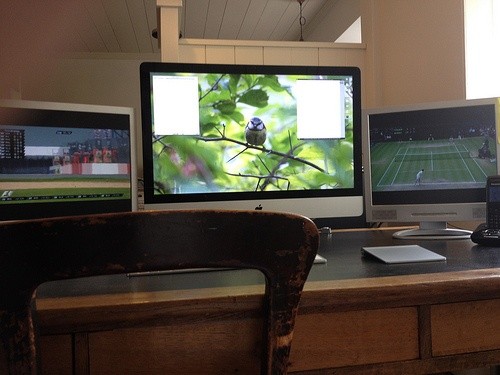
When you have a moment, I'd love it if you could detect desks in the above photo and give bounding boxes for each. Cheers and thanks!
[34,225,500,375]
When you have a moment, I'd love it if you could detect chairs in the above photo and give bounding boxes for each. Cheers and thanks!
[0,209,320,375]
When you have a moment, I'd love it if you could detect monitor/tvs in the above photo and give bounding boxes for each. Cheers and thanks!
[138,62,364,262]
[0,97,139,222]
[365,97,500,239]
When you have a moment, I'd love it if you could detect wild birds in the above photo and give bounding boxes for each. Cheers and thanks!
[245,117,266,153]
